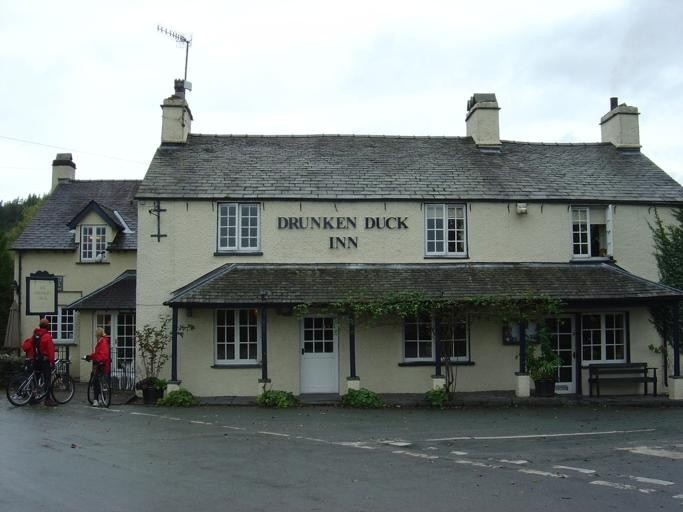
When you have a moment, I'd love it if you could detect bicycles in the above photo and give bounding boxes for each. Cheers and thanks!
[80,355,113,408]
[6,357,76,408]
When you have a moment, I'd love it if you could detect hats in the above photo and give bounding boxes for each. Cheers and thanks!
[40,319,48,327]
[95,327,106,337]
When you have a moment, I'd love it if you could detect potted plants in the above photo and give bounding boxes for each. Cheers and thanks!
[133,313,194,405]
[522,326,565,397]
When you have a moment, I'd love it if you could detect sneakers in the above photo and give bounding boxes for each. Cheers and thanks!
[93,400,99,406]
[45,399,58,405]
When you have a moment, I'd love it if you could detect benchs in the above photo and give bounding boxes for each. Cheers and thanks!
[587,362,658,399]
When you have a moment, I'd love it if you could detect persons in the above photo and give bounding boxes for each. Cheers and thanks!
[31,318,59,406]
[89,326,111,407]
[21,326,39,390]
